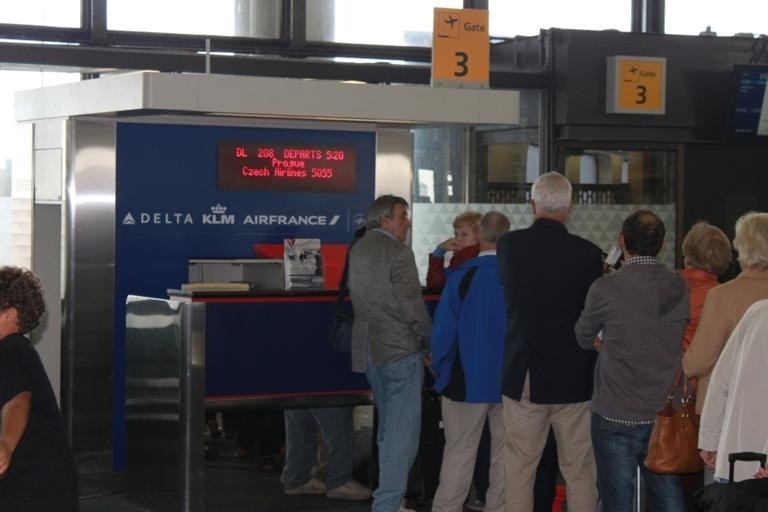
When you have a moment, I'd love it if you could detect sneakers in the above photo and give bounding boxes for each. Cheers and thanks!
[284,477,373,500]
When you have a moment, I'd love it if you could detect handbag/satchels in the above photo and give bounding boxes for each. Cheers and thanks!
[643,399,705,474]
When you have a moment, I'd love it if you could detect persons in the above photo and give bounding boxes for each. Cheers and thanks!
[0,266,78,512]
